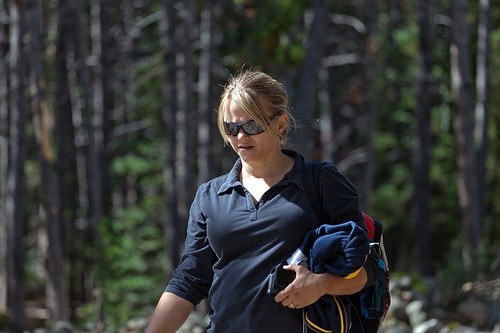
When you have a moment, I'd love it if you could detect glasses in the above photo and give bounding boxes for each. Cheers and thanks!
[221,108,282,137]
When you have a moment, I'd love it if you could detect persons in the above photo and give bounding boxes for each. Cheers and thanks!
[144,71,391,333]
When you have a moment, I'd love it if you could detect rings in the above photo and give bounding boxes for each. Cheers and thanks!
[290,303,296,309]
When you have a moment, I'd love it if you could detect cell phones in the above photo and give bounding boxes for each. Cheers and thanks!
[267,264,296,294]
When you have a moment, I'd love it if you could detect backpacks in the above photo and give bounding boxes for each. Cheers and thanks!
[302,157,389,333]
